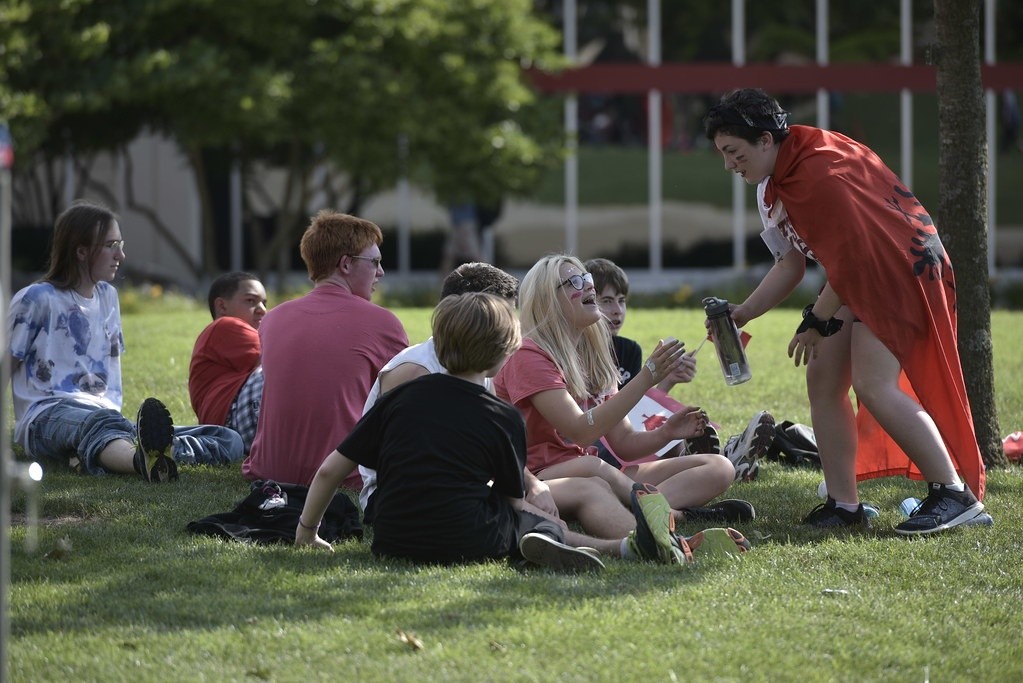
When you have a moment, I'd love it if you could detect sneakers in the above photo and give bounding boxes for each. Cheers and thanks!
[682,498,755,530]
[800,494,873,530]
[684,527,751,555]
[626,482,693,565]
[895,481,984,534]
[723,410,777,484]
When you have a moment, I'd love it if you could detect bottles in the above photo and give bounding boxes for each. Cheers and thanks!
[702,295,752,386]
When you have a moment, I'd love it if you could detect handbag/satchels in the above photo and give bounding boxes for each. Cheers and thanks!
[185,478,362,545]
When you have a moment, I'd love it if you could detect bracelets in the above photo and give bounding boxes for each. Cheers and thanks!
[299,515,321,528]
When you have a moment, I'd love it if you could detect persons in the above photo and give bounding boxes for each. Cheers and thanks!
[704,87,986,535]
[1,204,244,483]
[189,212,753,575]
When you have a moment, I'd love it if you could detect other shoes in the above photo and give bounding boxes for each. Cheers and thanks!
[130,397,187,484]
[519,531,606,575]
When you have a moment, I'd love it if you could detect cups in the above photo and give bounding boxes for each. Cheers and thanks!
[860,502,880,521]
[817,480,827,499]
[900,497,919,517]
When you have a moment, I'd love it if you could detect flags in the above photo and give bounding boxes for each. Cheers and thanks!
[708,327,752,348]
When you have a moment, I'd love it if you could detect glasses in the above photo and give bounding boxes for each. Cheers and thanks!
[557,272,594,290]
[335,253,380,269]
[93,240,125,250]
